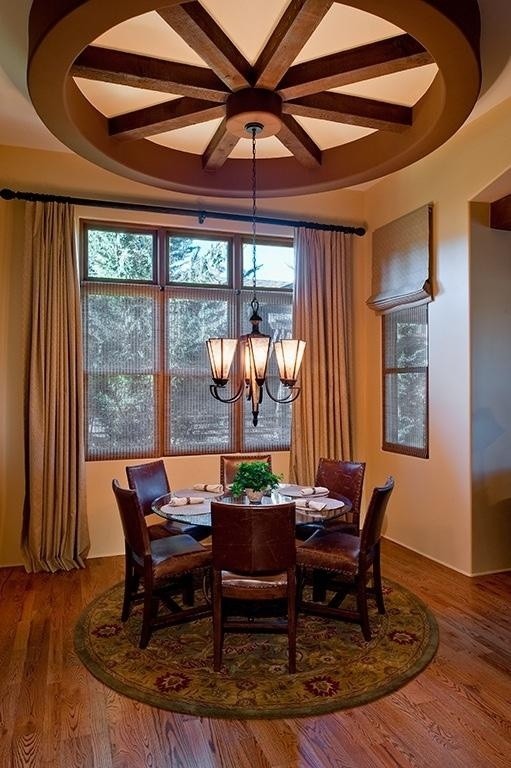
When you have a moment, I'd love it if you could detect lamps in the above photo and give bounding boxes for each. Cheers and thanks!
[206,122,307,427]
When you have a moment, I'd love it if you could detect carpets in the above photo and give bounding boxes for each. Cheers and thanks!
[73,571,439,717]
